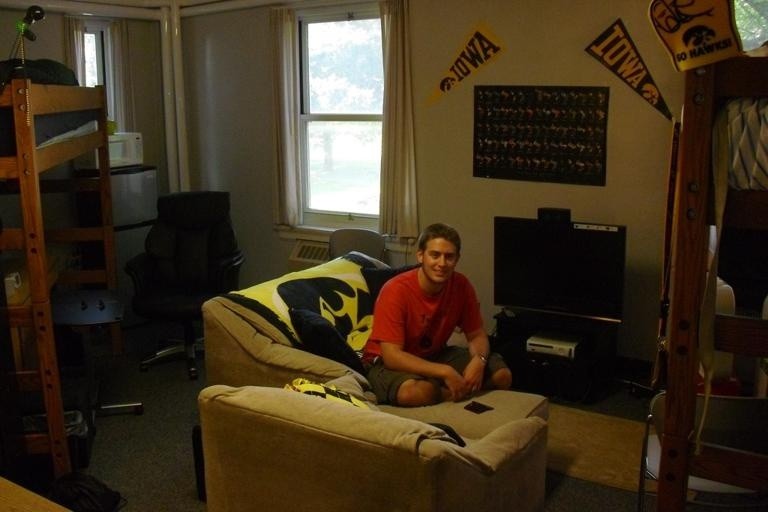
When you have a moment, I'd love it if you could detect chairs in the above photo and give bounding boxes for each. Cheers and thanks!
[326,229,389,264]
[120,187,248,380]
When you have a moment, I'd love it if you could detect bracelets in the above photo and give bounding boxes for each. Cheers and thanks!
[473,351,491,362]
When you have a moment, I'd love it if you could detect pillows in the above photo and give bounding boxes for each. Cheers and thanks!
[283,376,377,412]
[284,306,371,383]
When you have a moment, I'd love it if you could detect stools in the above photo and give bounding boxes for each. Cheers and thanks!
[26,284,147,435]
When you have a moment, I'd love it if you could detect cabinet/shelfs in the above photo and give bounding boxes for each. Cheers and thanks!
[491,303,622,406]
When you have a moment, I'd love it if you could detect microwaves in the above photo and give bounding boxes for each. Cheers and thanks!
[72,130,144,171]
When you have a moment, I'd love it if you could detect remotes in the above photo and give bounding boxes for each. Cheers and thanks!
[503,307,516,318]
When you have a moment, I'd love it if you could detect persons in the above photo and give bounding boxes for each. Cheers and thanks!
[362,222,515,408]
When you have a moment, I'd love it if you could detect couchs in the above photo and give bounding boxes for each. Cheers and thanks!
[193,374,550,510]
[201,250,481,403]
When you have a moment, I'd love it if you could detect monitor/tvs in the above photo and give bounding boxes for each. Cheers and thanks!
[493,215,627,328]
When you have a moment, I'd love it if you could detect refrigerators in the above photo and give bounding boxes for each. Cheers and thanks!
[72,166,160,329]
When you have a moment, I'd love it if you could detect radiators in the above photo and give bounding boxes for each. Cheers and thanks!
[287,240,390,275]
